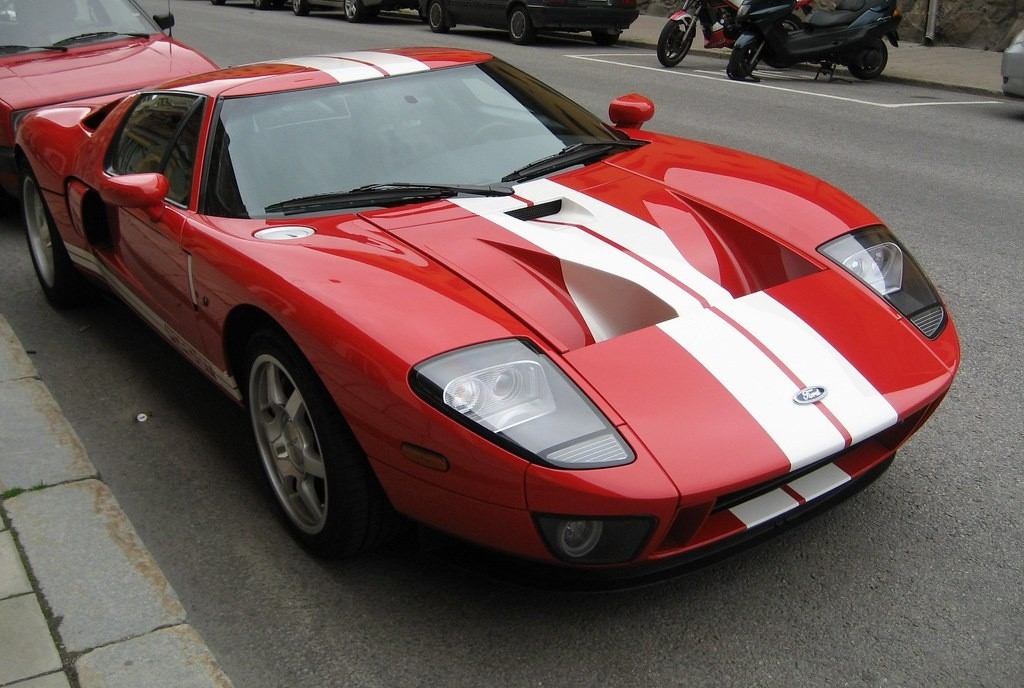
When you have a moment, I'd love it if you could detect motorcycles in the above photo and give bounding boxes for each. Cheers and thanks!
[725,0,903,83]
[657,0,817,67]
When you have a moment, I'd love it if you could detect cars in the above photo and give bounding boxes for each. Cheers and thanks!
[0,1,225,215]
[416,0,640,48]
[292,0,430,25]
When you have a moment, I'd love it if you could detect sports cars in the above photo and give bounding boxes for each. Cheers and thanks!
[13,44,959,570]
